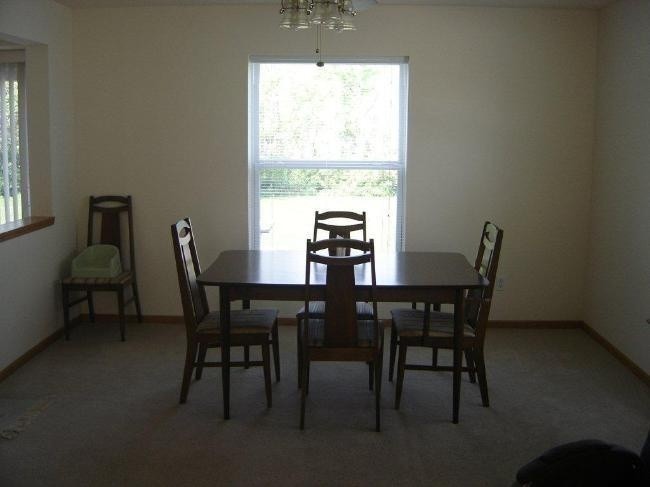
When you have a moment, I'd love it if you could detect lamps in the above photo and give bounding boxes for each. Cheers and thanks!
[278,1,362,66]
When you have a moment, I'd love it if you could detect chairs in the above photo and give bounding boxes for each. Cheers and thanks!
[57,194,144,341]
